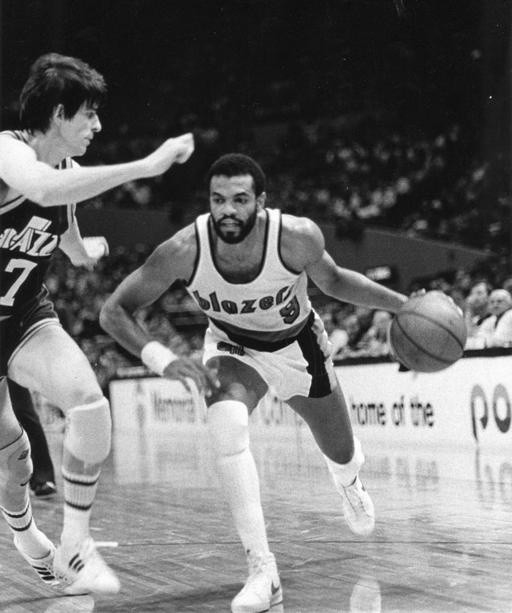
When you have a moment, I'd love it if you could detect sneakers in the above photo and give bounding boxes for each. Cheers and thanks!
[12,534,63,586]
[52,541,123,596]
[228,550,286,612]
[332,471,376,539]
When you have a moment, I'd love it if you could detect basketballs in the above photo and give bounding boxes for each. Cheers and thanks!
[389,294,466,371]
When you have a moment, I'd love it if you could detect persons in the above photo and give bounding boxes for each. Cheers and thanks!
[0,52,197,598]
[5,377,58,498]
[2,0,511,390]
[97,150,464,612]
